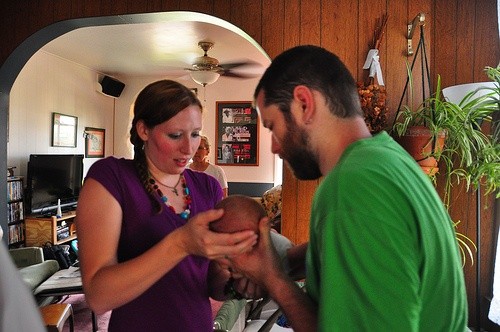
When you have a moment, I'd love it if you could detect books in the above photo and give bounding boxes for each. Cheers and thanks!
[6,180,25,244]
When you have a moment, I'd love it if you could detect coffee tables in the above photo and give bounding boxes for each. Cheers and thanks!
[34,267,98,332]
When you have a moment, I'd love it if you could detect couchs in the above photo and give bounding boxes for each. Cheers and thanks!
[8,247,60,290]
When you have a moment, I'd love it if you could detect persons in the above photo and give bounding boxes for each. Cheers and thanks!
[75,45,469,332]
[221,126,233,141]
[223,145,232,163]
[222,110,233,123]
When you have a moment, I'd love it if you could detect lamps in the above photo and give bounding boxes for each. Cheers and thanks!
[166,41,261,103]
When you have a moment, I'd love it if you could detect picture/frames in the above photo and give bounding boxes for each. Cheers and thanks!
[215,101,259,166]
[52,112,78,148]
[84,127,105,158]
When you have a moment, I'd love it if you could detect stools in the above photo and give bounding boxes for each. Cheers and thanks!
[40,303,74,332]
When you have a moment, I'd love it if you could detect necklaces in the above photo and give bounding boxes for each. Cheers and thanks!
[148,170,181,196]
[147,174,192,220]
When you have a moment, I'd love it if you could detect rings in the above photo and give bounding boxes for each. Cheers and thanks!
[254,298,263,303]
[244,291,254,302]
[224,255,228,259]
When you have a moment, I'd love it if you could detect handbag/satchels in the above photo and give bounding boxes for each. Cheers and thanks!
[42,241,72,270]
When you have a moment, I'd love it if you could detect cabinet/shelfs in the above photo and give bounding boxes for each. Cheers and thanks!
[24,210,78,248]
[7,176,25,250]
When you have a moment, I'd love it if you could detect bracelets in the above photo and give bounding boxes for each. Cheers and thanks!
[224,276,245,301]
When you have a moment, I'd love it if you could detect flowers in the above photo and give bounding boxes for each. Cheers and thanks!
[357,13,389,136]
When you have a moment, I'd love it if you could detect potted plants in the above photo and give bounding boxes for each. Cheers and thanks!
[392,61,500,270]
